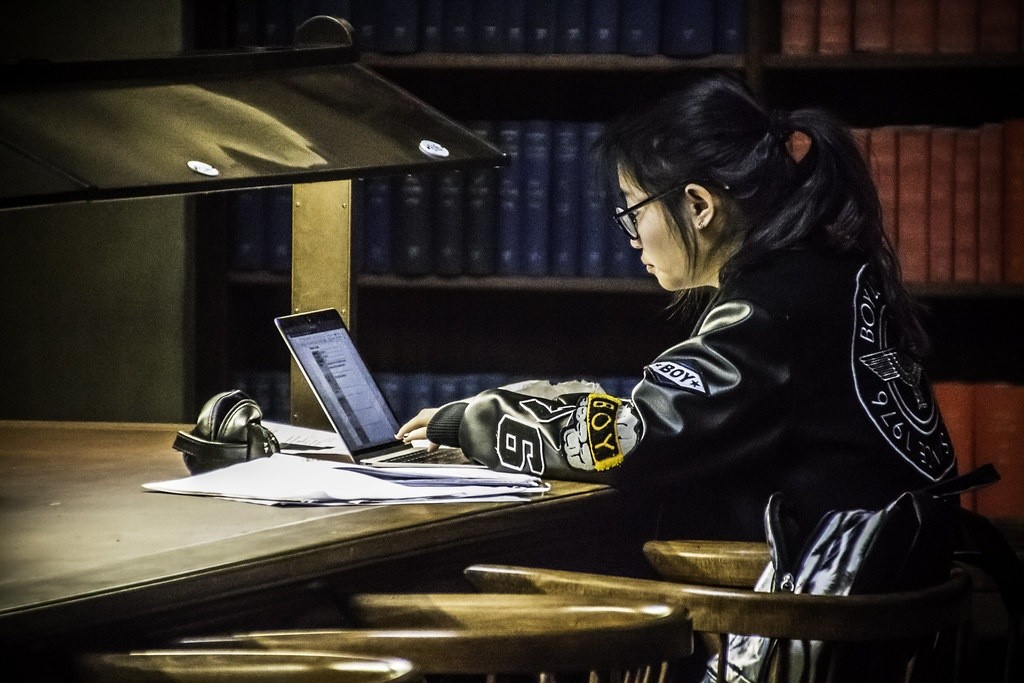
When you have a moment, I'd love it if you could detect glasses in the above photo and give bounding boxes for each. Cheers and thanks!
[612,176,729,240]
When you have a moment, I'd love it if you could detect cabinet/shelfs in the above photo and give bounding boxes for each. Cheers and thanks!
[191,0,1024,554]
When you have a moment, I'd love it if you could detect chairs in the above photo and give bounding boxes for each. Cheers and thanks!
[75,540,1014,683]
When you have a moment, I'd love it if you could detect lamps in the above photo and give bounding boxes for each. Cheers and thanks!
[0,15,510,211]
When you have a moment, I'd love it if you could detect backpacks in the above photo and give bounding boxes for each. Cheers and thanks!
[700,492,929,683]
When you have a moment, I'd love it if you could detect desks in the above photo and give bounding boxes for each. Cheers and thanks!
[0,420,624,664]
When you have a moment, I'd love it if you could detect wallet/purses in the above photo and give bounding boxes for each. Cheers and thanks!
[172,390,281,476]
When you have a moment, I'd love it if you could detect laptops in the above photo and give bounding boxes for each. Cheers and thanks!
[275,307,489,469]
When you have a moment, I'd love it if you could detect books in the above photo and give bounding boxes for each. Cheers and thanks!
[196,2,1023,521]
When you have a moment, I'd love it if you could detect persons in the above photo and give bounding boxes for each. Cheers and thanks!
[393,83,964,580]
